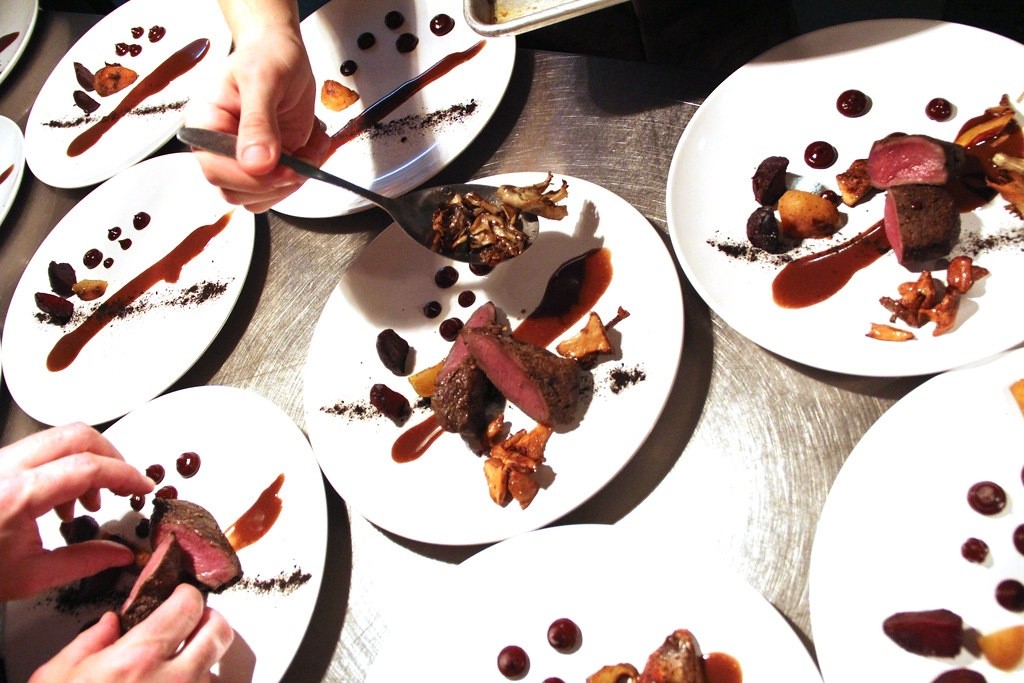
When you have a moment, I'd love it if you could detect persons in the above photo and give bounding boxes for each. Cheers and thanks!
[190,1,332,213]
[0,422,234,683]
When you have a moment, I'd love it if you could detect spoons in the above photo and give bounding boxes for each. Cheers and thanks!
[176,125,541,263]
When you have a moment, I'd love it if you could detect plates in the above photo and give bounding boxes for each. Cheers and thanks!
[2,3,41,81]
[807,350,1024,683]
[267,2,518,218]
[361,524,819,679]
[1,151,256,429]
[19,388,326,679]
[25,2,233,188]
[665,19,1021,376]
[301,173,681,547]
[1,112,25,227]
